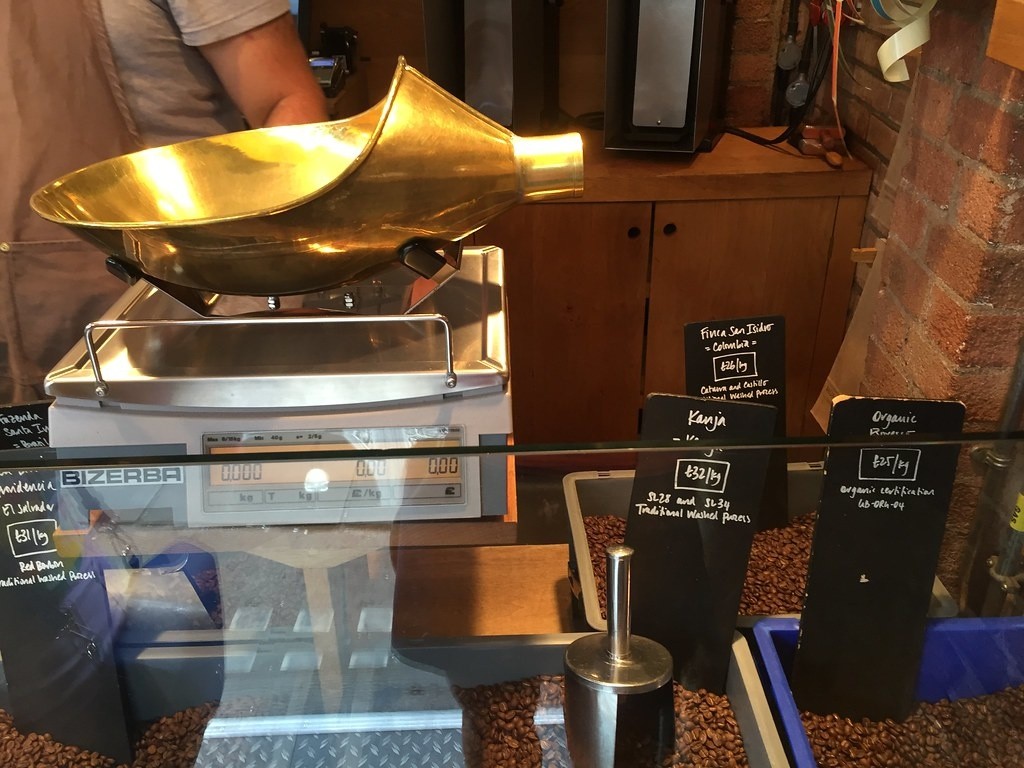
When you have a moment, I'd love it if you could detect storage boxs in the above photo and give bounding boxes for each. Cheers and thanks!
[396,631,790,768]
[561,460,960,630]
[751,614,1024,768]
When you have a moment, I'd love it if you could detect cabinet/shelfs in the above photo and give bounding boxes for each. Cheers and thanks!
[475,125,876,470]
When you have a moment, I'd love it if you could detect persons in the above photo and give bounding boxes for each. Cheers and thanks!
[100,0,330,148]
